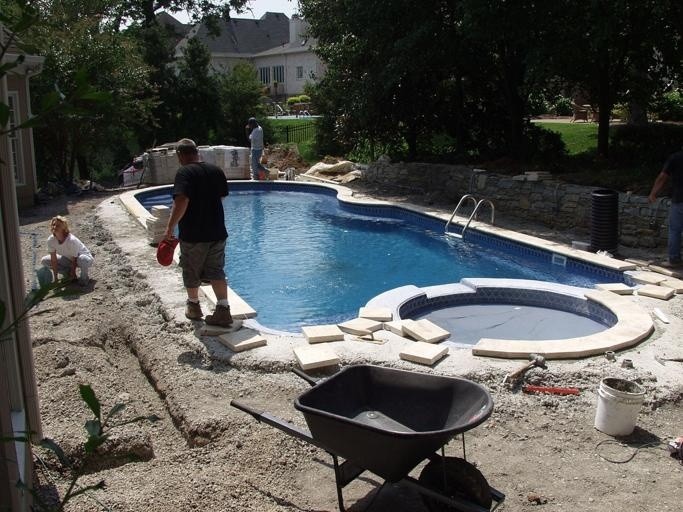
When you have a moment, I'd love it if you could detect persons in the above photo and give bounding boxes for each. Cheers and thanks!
[41,216,94,287]
[246,117,271,180]
[648,151,683,266]
[164,138,234,325]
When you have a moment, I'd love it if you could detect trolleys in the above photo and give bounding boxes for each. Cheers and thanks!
[230,363,505,511]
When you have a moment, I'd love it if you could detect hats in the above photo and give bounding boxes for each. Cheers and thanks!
[157,239,177,266]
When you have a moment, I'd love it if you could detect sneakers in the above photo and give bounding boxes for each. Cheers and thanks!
[186,302,200,320]
[204,306,232,327]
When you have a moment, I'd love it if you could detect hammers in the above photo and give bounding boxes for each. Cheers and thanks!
[521,385,579,396]
[508,351,546,380]
[654,352,683,367]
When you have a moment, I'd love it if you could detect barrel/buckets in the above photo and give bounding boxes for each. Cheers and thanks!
[595,378,645,436]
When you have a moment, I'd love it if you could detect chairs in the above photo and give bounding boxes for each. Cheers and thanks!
[570,101,589,123]
